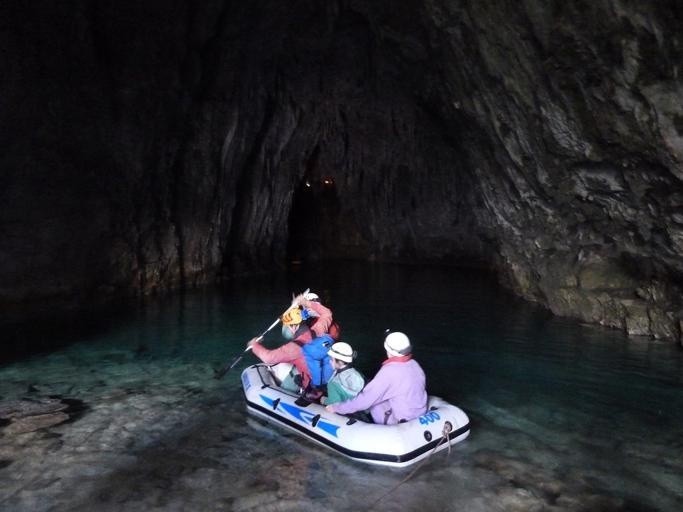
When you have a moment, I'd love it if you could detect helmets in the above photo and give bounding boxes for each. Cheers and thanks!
[383,331,411,357]
[327,341,354,363]
[281,308,303,325]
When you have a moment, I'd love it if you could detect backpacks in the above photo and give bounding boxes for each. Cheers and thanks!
[292,329,336,388]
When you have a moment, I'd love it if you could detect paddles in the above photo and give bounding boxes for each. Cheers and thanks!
[215,287,310,380]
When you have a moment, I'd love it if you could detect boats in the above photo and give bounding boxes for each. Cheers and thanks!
[238,361,472,471]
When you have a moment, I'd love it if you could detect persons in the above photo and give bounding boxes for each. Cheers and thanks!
[247,293,341,402]
[325,332,428,425]
[320,341,365,406]
